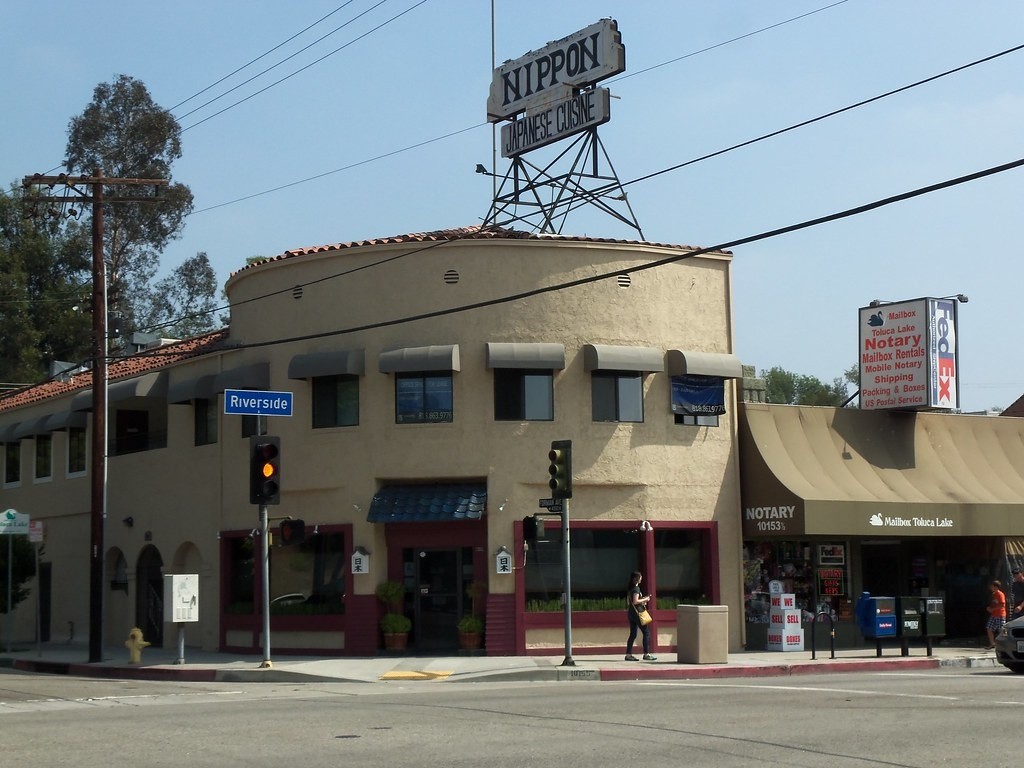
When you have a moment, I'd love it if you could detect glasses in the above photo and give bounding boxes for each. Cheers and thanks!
[1014,572,1019,574]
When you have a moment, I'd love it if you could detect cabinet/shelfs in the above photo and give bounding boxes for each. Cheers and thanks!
[819,568,844,612]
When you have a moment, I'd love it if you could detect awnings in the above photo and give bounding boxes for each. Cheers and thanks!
[485,342,565,370]
[288,347,364,380]
[738,402,1024,538]
[44,409,86,431]
[0,421,20,442]
[168,361,269,405]
[583,344,664,372]
[70,371,168,411]
[378,344,460,373]
[667,349,743,379]
[13,414,50,438]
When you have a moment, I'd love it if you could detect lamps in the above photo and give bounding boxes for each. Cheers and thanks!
[941,294,968,303]
[870,299,891,307]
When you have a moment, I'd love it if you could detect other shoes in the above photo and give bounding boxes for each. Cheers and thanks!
[985,645,995,650]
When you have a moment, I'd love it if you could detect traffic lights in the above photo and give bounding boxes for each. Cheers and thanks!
[548,439,572,499]
[523,517,545,542]
[249,435,281,505]
[280,518,305,544]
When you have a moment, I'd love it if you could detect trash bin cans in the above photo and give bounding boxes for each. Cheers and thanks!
[677,605,728,664]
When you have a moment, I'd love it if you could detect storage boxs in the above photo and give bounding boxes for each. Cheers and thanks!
[767,593,804,650]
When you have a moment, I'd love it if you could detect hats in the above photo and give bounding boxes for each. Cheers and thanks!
[1012,567,1024,572]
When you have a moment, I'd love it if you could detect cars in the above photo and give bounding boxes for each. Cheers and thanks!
[995,615,1024,673]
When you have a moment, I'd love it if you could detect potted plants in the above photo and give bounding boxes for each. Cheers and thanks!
[457,582,483,647]
[374,581,411,654]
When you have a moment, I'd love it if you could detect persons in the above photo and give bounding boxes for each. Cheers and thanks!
[625,571,658,661]
[1011,568,1024,620]
[985,580,1007,648]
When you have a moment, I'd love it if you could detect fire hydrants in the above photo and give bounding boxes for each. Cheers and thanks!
[125,627,150,663]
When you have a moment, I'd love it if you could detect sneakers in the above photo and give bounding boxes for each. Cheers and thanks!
[643,655,657,660]
[625,656,639,661]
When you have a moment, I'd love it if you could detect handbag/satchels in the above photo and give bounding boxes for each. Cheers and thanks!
[639,611,652,626]
